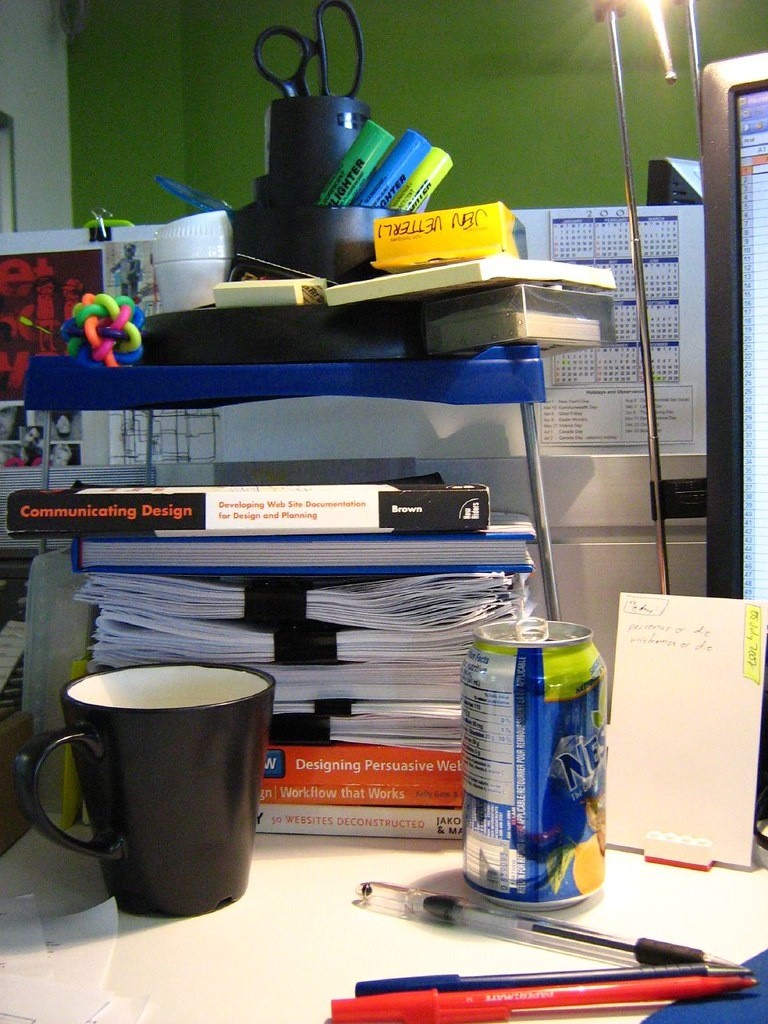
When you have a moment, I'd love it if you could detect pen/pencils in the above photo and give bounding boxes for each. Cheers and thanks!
[330,880,760,1024]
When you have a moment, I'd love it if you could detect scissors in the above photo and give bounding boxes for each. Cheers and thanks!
[254,0,364,99]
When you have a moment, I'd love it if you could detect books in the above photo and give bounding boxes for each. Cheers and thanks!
[73,569,542,843]
[5,472,495,538]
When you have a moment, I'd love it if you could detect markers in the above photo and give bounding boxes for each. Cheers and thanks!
[317,119,454,211]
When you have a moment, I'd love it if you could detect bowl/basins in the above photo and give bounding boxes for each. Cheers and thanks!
[232,207,409,286]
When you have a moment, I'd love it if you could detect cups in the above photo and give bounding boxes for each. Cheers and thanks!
[13,662,276,918]
[266,96,370,206]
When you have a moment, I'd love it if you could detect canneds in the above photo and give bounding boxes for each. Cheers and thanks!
[460,620,608,911]
